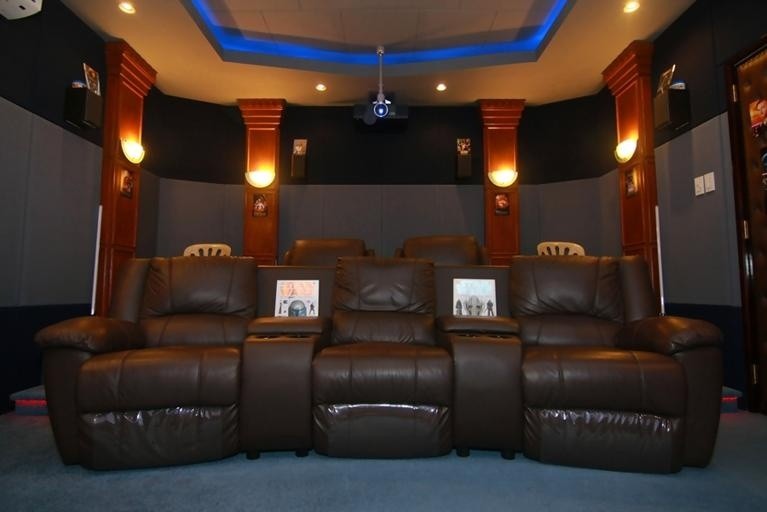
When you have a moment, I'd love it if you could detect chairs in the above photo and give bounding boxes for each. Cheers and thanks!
[394,232,491,268]
[31,254,259,474]
[536,239,588,258]
[277,236,373,266]
[181,240,233,256]
[308,251,458,464]
[507,248,728,475]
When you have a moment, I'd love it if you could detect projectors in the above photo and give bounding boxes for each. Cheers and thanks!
[355,101,410,120]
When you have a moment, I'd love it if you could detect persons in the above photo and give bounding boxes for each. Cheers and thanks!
[308,302,315,316]
[455,300,462,316]
[486,300,494,317]
[255,199,264,210]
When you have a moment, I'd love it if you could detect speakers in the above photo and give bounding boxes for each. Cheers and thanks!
[291,155,307,178]
[457,154,473,178]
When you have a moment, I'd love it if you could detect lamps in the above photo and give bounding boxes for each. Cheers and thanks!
[120,137,146,167]
[242,169,277,190]
[485,168,520,188]
[612,137,644,164]
